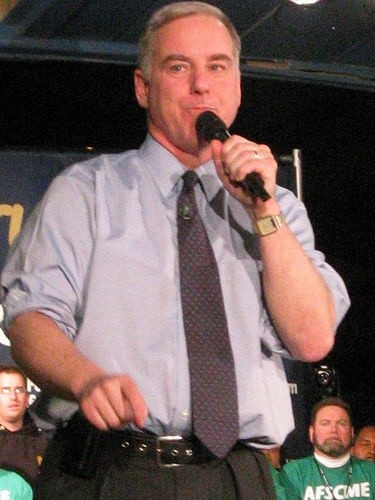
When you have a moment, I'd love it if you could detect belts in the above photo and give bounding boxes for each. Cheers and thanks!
[110,429,216,469]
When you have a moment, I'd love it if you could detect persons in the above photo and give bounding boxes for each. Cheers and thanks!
[350,415,375,461]
[1,0,351,500]
[261,448,285,471]
[0,366,48,500]
[269,398,375,499]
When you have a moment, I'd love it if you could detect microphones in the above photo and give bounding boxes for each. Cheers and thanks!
[195,110,271,201]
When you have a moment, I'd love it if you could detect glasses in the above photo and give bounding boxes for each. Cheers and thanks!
[0,388,28,394]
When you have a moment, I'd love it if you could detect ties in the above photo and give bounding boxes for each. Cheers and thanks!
[176,171,239,459]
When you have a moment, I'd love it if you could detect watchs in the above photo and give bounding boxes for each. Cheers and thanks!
[250,211,286,237]
